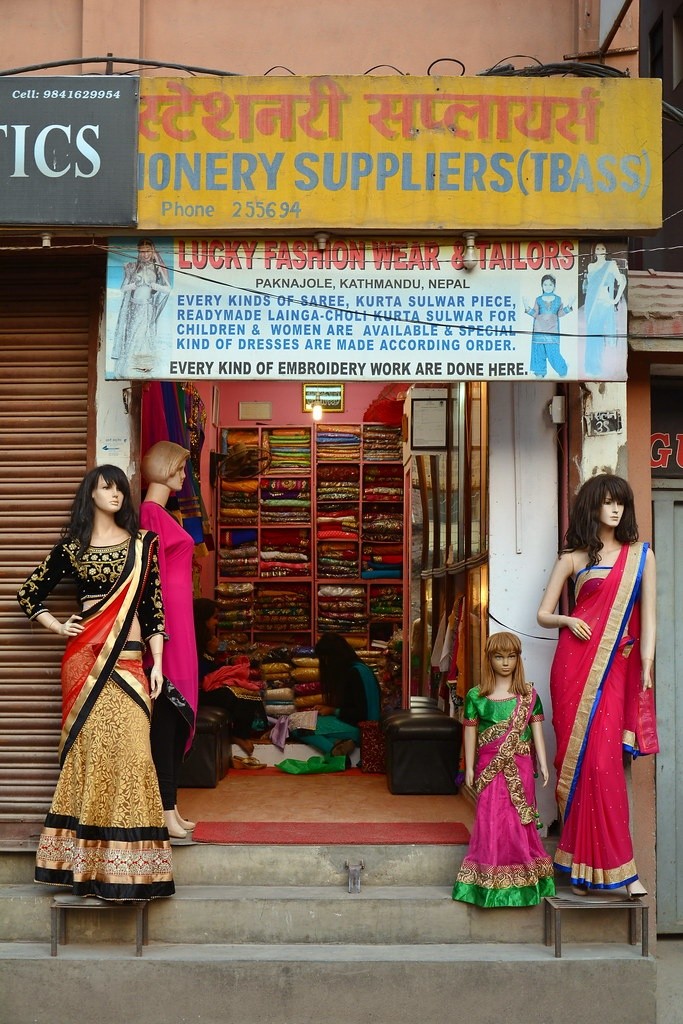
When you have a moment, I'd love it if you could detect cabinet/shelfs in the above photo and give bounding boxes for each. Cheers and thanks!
[215,421,406,680]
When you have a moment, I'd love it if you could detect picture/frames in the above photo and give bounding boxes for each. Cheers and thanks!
[302,383,344,413]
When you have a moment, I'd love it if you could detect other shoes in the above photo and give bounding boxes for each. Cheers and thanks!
[331,739,355,756]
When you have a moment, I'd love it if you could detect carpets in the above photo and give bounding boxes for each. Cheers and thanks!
[192,819,470,846]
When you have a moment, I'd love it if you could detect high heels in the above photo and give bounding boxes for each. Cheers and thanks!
[232,754,267,769]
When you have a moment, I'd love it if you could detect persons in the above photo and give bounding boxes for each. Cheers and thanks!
[581,241,626,378]
[193,597,268,758]
[536,474,657,899]
[450,631,556,908]
[110,237,171,379]
[522,274,576,380]
[139,440,200,839]
[16,463,176,903]
[290,631,380,758]
